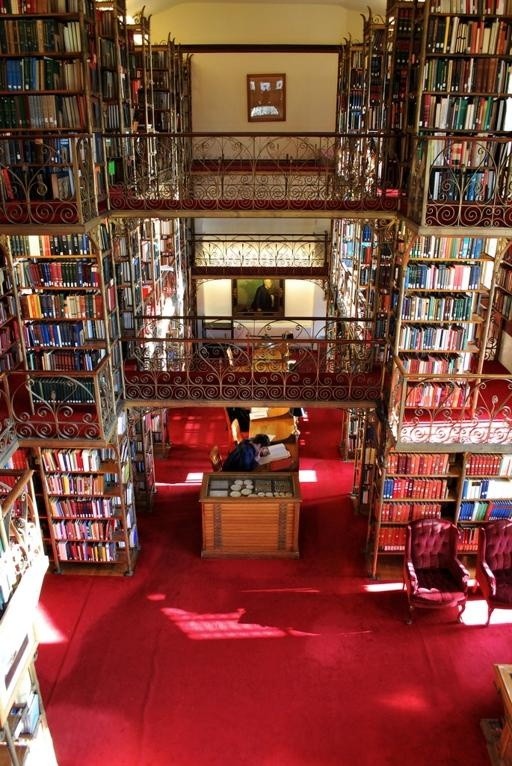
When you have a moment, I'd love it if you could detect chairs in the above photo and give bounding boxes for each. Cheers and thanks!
[208,407,248,473]
[400,518,512,626]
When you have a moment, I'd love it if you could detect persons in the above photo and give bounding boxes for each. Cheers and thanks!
[249,279,277,316]
[221,432,271,472]
[235,407,251,434]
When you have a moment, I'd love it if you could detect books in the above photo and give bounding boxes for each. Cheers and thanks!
[248,407,268,420]
[0,668,40,742]
[256,442,292,466]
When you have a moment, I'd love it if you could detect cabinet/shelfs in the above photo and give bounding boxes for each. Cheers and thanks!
[330,219,512,420]
[0,446,58,766]
[1,0,194,200]
[202,320,233,358]
[198,472,303,558]
[38,410,171,577]
[334,0,512,202]
[0,216,198,415]
[339,406,512,578]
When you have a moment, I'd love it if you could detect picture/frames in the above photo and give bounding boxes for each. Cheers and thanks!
[247,73,286,122]
[231,278,285,320]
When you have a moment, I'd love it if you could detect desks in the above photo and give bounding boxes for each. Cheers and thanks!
[249,407,299,472]
